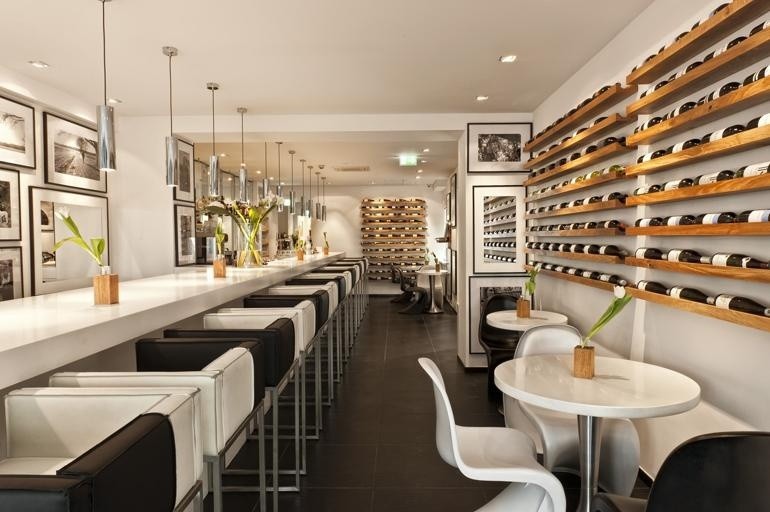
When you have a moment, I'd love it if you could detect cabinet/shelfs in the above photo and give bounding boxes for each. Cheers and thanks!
[362,198,427,280]
[483,196,518,264]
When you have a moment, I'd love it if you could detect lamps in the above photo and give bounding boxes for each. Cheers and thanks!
[161,48,181,187]
[237,107,248,202]
[207,83,220,197]
[96,0,118,173]
[263,142,328,222]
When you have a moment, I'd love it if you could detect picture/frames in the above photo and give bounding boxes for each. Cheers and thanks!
[467,273,535,355]
[445,248,458,300]
[173,137,198,268]
[0,94,110,302]
[445,172,458,227]
[467,122,532,173]
[194,159,254,216]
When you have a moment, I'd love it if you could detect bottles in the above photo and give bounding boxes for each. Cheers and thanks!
[359,198,426,279]
[526,1,769,318]
[483,198,518,263]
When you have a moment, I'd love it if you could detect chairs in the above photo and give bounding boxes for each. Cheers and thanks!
[390,263,428,317]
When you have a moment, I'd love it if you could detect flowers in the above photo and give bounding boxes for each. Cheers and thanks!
[200,193,282,266]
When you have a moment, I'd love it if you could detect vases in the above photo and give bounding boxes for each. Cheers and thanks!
[235,223,264,270]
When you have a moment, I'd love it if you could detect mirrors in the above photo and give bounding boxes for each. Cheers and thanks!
[474,184,529,275]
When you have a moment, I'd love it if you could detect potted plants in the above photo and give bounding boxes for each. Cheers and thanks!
[212,223,225,277]
